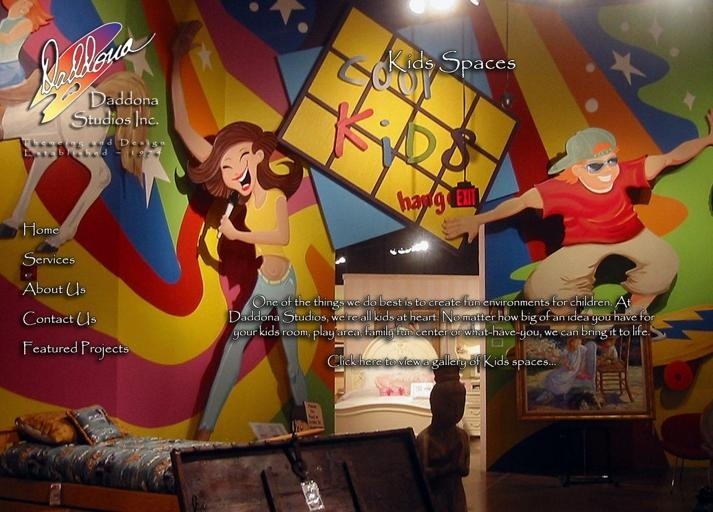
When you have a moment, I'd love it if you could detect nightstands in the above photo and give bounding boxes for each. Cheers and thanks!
[460,379,481,442]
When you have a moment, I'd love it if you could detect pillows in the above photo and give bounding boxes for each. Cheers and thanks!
[357,369,435,398]
[66,404,126,448]
[15,408,83,446]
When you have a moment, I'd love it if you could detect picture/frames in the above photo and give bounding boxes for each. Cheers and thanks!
[274,2,522,259]
[515,318,656,421]
[335,343,344,373]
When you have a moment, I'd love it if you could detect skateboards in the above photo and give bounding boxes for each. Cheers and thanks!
[648,304,713,392]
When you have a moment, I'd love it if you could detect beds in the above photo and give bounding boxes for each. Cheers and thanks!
[334,327,463,446]
[0,414,239,512]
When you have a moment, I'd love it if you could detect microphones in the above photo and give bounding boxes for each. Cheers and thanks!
[216,192,238,238]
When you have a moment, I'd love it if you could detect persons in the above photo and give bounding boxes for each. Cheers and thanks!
[595,334,619,366]
[412,358,470,511]
[533,335,588,404]
[0,0,55,144]
[441,107,712,355]
[166,18,314,441]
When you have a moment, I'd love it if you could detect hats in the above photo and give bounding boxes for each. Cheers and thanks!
[546,127,617,173]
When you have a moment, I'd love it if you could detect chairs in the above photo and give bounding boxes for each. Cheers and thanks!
[661,412,713,504]
[574,341,602,409]
[596,332,635,403]
